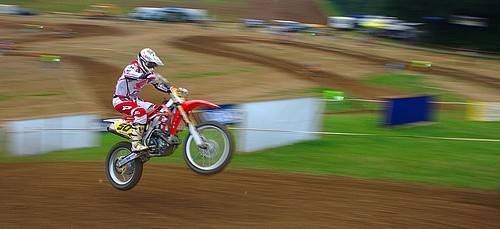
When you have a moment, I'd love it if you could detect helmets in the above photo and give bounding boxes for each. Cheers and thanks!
[137,48,164,74]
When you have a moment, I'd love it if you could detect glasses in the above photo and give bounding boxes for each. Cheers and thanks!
[146,62,156,68]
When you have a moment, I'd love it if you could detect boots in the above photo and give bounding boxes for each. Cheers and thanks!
[130,122,149,152]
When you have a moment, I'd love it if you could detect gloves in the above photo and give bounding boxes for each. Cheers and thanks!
[144,73,153,79]
[177,87,189,95]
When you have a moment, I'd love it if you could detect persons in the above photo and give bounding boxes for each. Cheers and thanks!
[110,48,187,151]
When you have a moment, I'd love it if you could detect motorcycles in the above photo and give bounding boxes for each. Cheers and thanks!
[101,73,233,191]
